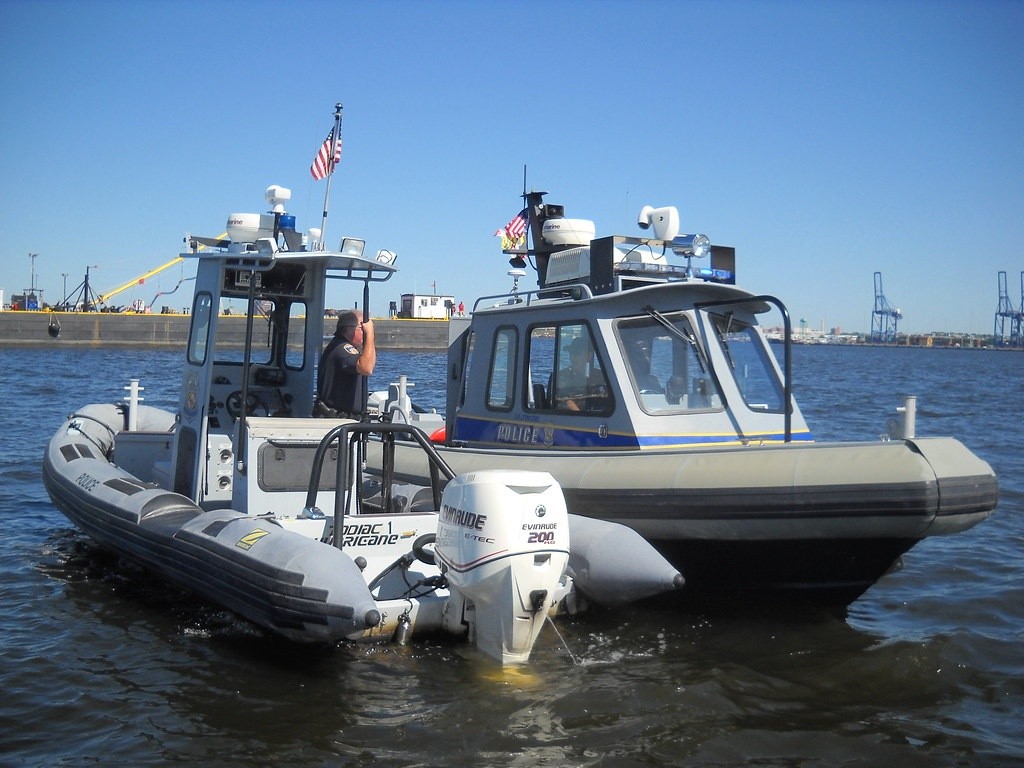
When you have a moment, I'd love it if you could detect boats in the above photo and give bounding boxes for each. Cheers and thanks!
[40,103,688,665]
[354,164,999,592]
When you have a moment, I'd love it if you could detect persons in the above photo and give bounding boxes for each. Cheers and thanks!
[451,302,465,317]
[314,310,376,420]
[553,337,605,411]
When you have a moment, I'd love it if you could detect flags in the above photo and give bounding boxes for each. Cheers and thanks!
[503,209,530,240]
[309,117,343,181]
